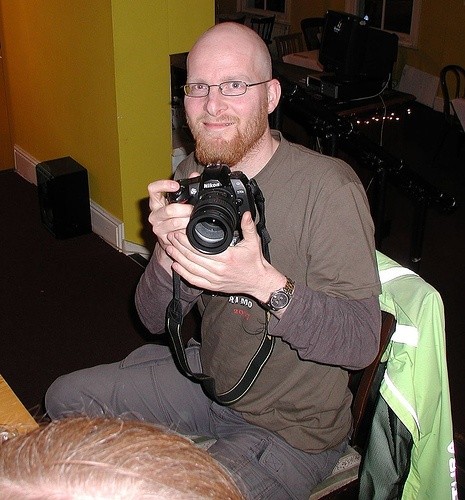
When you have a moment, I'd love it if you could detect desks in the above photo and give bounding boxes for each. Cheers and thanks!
[271,63,417,158]
[282,50,324,73]
[351,109,465,273]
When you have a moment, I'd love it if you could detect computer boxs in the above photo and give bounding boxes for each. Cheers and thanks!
[306,73,379,100]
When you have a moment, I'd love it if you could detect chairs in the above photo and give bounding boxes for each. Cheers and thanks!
[441,64,465,117]
[217,16,322,61]
[309,250,441,499]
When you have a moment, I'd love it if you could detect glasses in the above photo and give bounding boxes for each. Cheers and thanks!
[182,79,273,98]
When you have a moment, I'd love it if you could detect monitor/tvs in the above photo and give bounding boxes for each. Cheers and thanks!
[318,10,367,84]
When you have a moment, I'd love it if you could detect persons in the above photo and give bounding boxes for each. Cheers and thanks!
[0,412,245,500]
[45,19,384,500]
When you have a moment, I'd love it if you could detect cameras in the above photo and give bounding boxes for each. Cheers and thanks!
[168,160,264,257]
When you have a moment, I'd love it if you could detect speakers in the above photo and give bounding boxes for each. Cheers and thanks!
[35,157,92,240]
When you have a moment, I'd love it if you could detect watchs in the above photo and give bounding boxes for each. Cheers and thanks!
[259,276,297,311]
